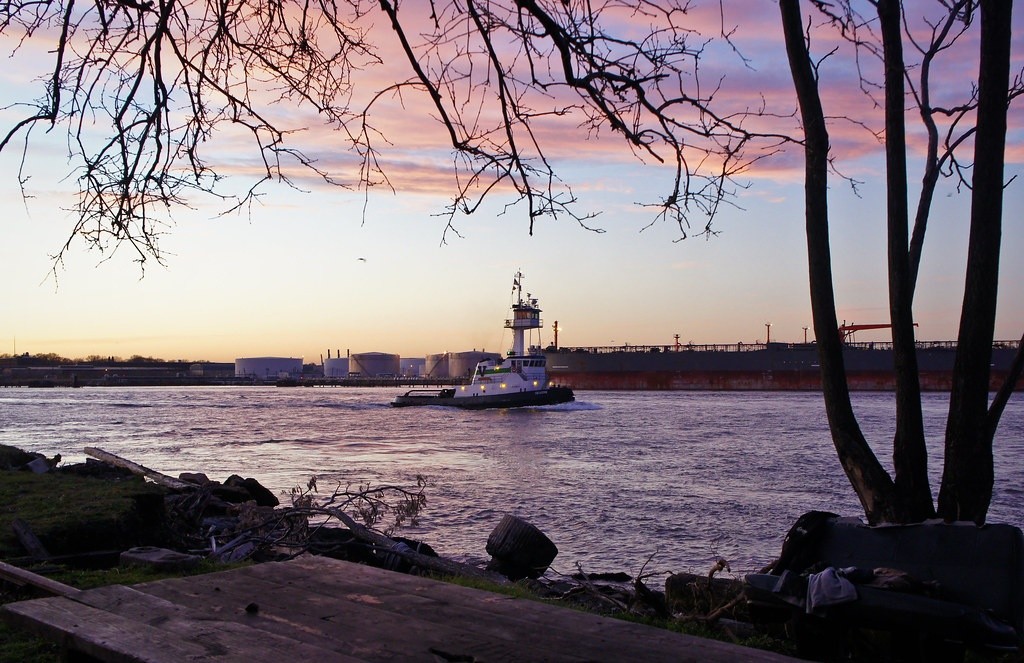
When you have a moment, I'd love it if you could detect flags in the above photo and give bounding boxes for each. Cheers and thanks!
[512,286,516,290]
[514,278,519,285]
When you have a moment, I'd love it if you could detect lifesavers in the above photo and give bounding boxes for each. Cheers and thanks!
[678,343,681,347]
[512,368,517,372]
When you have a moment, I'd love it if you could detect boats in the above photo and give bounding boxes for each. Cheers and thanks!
[391,267,576,409]
